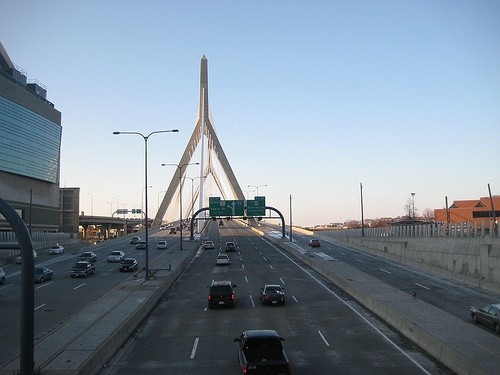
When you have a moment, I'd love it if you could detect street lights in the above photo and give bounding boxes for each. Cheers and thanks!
[87,185,170,219]
[113,129,179,280]
[241,184,269,198]
[161,161,200,251]
[411,191,416,221]
[180,176,206,229]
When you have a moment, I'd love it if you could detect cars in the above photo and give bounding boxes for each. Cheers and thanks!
[308,239,321,248]
[260,283,285,306]
[0,219,237,291]
[470,303,500,335]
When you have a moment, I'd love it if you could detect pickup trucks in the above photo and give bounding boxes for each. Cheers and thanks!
[234,331,289,375]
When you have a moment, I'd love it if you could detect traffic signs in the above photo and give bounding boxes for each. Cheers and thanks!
[209,197,244,215]
[247,196,265,216]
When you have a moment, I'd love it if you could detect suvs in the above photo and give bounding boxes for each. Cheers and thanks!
[206,280,237,308]
